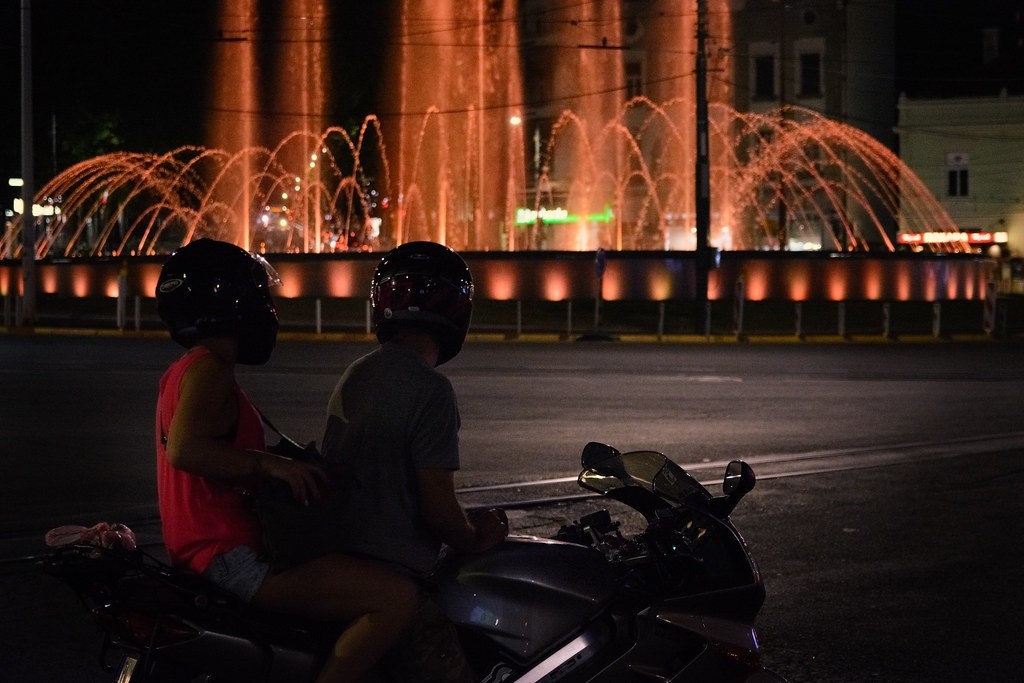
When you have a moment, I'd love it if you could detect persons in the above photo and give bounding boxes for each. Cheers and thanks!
[323,243,508,683]
[155,244,418,683]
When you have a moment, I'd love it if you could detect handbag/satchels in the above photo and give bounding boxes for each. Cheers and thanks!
[244,390,334,563]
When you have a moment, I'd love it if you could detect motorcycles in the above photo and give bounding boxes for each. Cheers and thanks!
[1,442,790,683]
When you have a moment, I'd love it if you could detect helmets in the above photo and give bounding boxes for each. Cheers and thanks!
[365,241,475,366]
[154,238,283,368]
